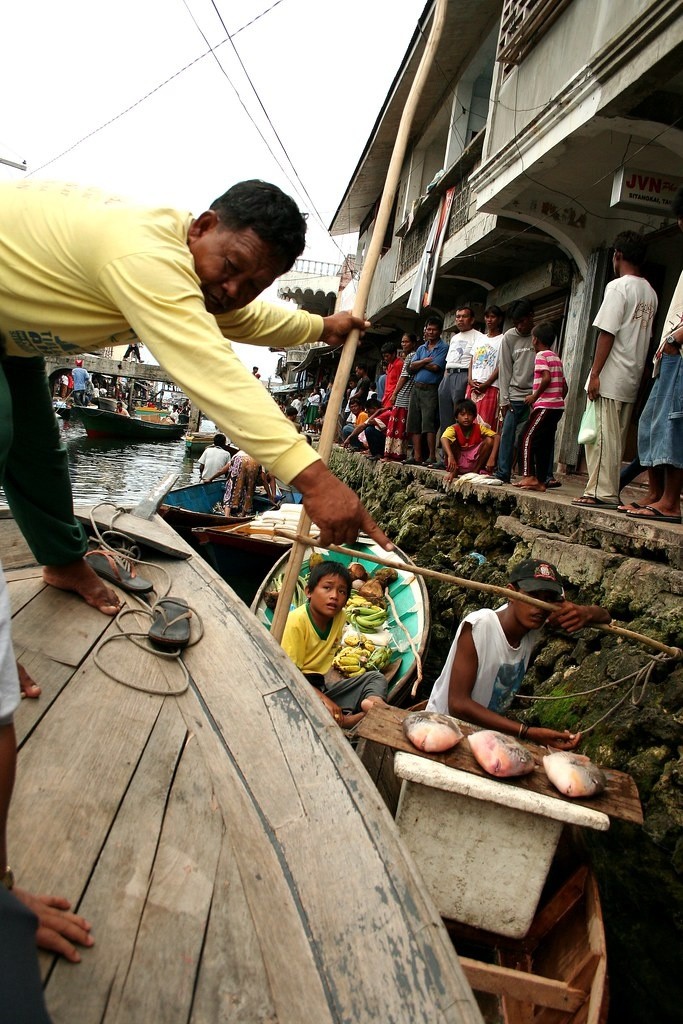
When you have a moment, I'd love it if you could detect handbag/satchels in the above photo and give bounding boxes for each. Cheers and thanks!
[578,398,597,447]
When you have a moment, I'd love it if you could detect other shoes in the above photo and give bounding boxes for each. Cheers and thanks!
[545,477,562,487]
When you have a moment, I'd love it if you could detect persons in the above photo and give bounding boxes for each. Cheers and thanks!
[53,301,483,527]
[464,305,507,474]
[440,397,501,485]
[495,304,562,488]
[0,568,100,1024]
[569,228,661,507]
[0,175,395,704]
[424,555,613,752]
[274,560,394,730]
[512,321,566,494]
[608,267,683,524]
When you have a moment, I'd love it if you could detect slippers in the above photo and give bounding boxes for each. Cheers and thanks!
[617,502,640,512]
[570,495,624,509]
[83,546,153,594]
[148,596,192,644]
[401,456,422,465]
[421,458,437,466]
[626,505,682,523]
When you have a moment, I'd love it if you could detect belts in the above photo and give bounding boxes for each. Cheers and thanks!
[412,382,436,390]
[446,368,469,373]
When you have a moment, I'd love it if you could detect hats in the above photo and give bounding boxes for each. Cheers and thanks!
[507,559,563,595]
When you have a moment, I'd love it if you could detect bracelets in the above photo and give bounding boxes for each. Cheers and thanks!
[517,723,530,741]
[4,864,14,892]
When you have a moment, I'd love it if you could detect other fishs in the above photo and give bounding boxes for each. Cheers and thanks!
[543,744,608,799]
[454,472,504,487]
[401,711,464,753]
[467,729,541,778]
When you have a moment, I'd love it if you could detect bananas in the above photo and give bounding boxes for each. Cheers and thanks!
[331,595,394,678]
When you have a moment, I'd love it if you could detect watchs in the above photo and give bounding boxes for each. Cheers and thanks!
[666,335,681,349]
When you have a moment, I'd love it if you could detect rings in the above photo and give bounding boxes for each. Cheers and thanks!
[334,713,340,719]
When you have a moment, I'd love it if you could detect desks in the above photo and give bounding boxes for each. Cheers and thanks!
[359,699,642,940]
